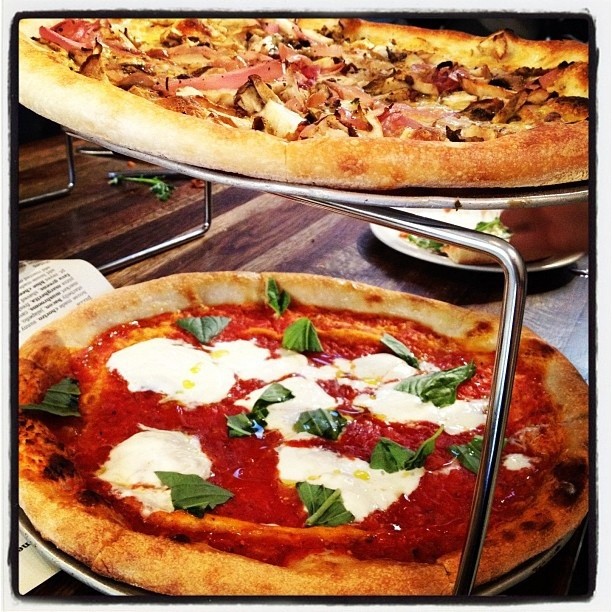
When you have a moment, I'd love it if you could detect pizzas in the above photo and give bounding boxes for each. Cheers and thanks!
[19,18,588,191]
[18,271,589,596]
[399,199,592,265]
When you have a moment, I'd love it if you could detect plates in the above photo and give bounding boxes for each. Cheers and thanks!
[369,208,587,273]
